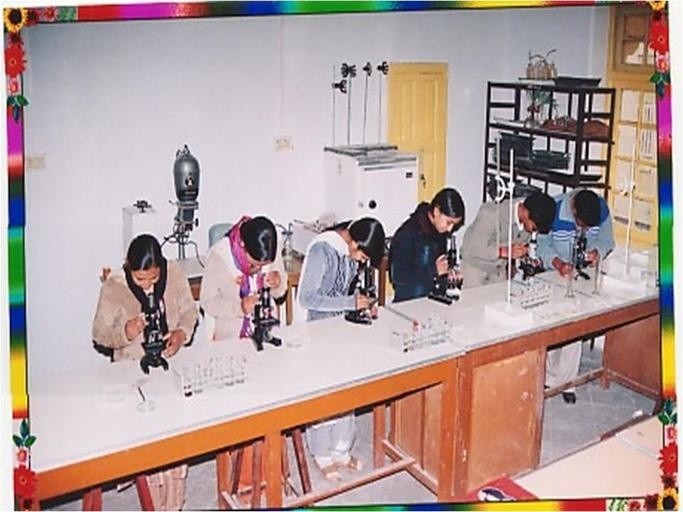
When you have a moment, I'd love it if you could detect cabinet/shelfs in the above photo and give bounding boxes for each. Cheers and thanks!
[477,76,614,226]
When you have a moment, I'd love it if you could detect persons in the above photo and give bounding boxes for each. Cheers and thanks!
[92,234,197,511]
[196,216,289,500]
[535,189,616,404]
[389,187,464,303]
[295,218,384,482]
[458,190,556,289]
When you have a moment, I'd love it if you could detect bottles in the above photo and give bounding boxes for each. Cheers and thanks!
[526,63,557,78]
[283,236,294,271]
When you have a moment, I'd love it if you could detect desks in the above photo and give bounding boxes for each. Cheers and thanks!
[30,268,664,509]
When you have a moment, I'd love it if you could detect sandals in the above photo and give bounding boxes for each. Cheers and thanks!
[326,456,362,483]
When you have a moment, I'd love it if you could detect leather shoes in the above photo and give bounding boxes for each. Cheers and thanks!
[562,392,576,404]
